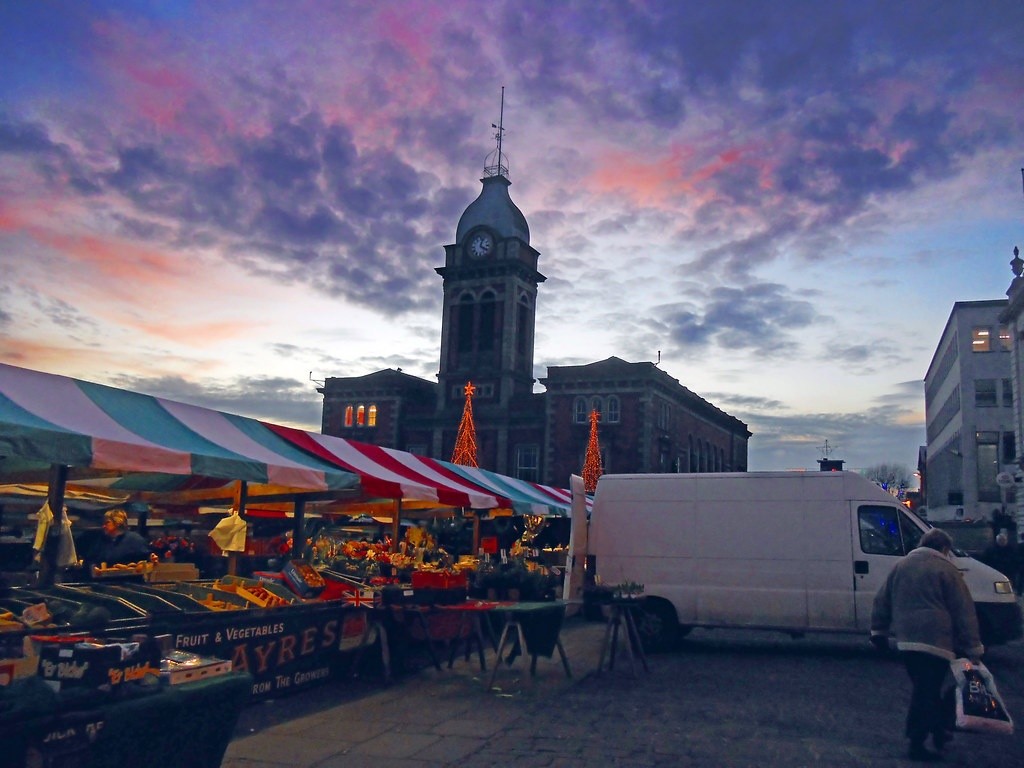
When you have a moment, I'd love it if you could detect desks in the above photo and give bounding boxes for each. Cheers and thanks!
[392,600,574,702]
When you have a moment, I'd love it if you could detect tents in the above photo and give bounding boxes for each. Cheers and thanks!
[0,361,594,569]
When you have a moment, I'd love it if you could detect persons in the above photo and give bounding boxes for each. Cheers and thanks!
[85,509,151,564]
[868,529,984,760]
[986,531,1024,597]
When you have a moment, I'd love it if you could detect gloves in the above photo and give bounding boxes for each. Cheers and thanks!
[869,635,898,663]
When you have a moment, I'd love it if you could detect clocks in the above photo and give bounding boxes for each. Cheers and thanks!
[461,224,496,263]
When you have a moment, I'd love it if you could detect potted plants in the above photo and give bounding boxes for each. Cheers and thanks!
[586,582,644,619]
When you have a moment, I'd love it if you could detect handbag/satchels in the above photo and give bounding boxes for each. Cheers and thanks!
[950,657,1013,736]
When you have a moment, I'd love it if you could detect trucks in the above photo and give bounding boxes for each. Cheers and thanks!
[587,470,1023,653]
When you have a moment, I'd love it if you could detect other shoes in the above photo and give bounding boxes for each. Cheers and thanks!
[931,730,954,750]
[907,744,945,762]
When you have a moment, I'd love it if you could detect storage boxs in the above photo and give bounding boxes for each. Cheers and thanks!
[283,559,327,598]
[254,570,286,586]
[411,571,467,588]
[144,562,199,582]
